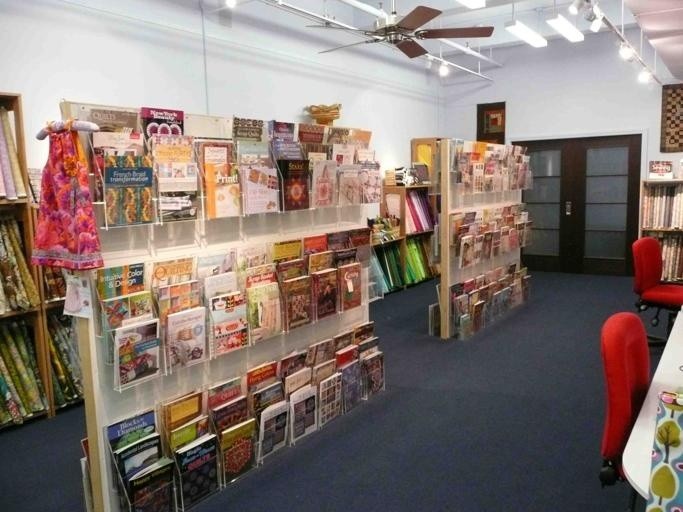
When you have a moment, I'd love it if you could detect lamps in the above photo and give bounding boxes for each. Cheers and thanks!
[619,47,633,62]
[546,0,584,42]
[590,16,603,33]
[425,57,434,69]
[569,0,584,16]
[456,0,486,9]
[638,69,652,83]
[439,62,449,77]
[504,0,547,48]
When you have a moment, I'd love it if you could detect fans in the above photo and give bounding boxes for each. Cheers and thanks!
[305,0,494,58]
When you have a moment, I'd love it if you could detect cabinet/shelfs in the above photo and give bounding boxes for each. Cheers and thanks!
[371,184,436,295]
[441,138,522,339]
[0,91,51,431]
[411,137,441,277]
[60,102,369,512]
[638,179,683,284]
[31,203,85,418]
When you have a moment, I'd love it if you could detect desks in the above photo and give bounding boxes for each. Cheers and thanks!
[623,304,683,500]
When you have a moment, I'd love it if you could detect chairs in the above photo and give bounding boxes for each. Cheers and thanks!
[633,237,683,346]
[599,312,650,512]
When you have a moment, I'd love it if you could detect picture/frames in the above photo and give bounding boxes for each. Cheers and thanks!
[660,83,683,152]
[477,102,505,145]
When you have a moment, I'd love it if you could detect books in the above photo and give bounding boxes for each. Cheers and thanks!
[94,148,137,168]
[338,262,361,312]
[313,359,336,385]
[308,250,333,275]
[339,167,362,206]
[290,385,317,444]
[363,351,385,398]
[158,162,198,221]
[356,150,376,165]
[429,304,440,336]
[171,413,209,451]
[206,164,242,219]
[450,204,533,270]
[303,234,327,256]
[151,255,196,287]
[319,373,342,427]
[248,360,281,392]
[308,152,326,170]
[80,437,93,512]
[245,282,283,345]
[275,141,303,160]
[340,359,367,415]
[166,306,206,368]
[158,280,200,326]
[278,159,309,211]
[96,260,146,299]
[114,319,160,387]
[78,105,138,133]
[207,377,242,410]
[450,138,533,195]
[280,350,307,379]
[152,144,191,162]
[232,116,269,146]
[209,291,249,360]
[252,381,286,419]
[307,338,336,366]
[311,268,338,323]
[235,243,270,275]
[335,344,358,368]
[333,247,357,268]
[102,289,152,330]
[105,167,154,226]
[103,408,157,493]
[351,129,371,150]
[203,272,236,306]
[327,231,349,251]
[243,263,276,288]
[140,107,184,144]
[175,433,222,507]
[258,400,289,461]
[112,433,162,512]
[220,417,257,484]
[284,367,311,400]
[92,132,144,160]
[298,123,327,143]
[162,390,203,429]
[354,321,375,344]
[436,284,440,301]
[213,394,249,432]
[332,143,354,166]
[311,160,337,208]
[348,228,372,248]
[242,166,280,216]
[280,275,311,330]
[450,263,532,341]
[270,238,302,265]
[276,259,305,281]
[360,166,382,204]
[358,337,378,360]
[328,127,350,145]
[334,330,355,351]
[197,250,235,279]
[273,122,293,146]
